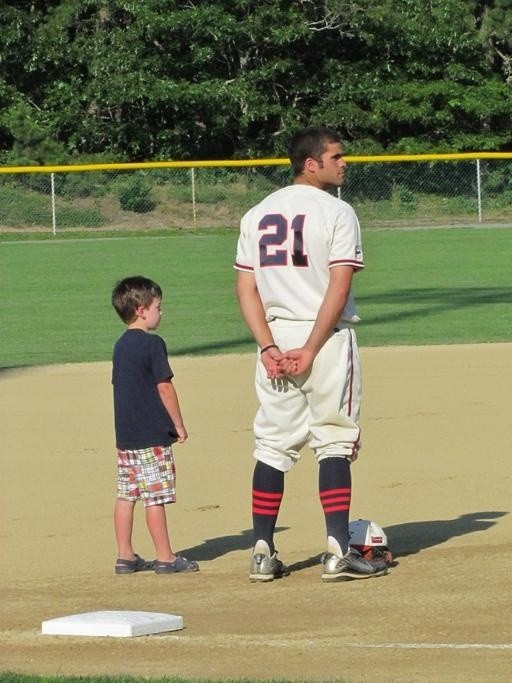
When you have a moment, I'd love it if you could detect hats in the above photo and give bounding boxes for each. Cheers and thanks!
[348,519,387,553]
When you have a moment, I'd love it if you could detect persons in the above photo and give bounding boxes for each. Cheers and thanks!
[233,125,388,579]
[112,277,200,572]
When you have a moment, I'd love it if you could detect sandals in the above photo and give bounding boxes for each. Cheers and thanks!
[116,553,156,574]
[155,554,200,574]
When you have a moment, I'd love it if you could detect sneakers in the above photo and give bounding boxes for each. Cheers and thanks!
[250,552,289,581]
[321,547,388,581]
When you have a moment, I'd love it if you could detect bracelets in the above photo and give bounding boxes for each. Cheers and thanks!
[257,342,280,353]
